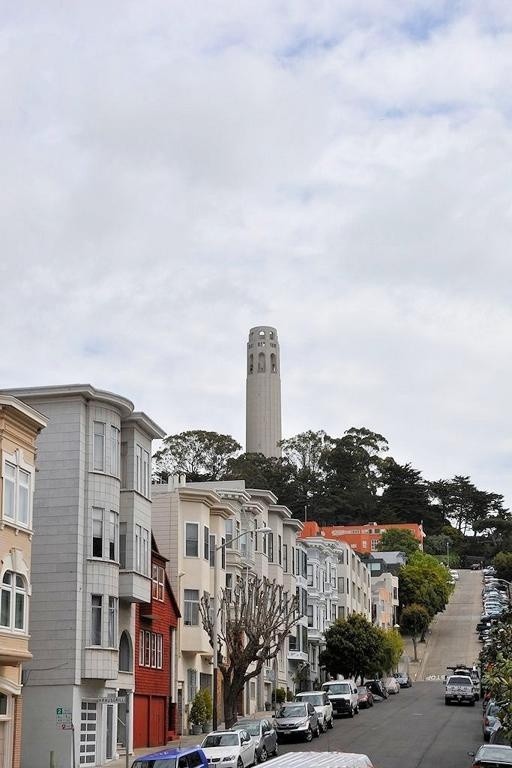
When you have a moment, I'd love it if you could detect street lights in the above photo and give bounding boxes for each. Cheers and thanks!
[446,542,449,566]
[393,624,401,672]
[212,527,273,730]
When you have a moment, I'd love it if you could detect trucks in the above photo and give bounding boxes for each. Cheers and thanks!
[249,751,374,767]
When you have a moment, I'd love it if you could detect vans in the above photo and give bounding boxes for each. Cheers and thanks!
[319,680,360,718]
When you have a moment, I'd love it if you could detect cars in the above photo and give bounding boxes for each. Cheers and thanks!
[198,729,258,768]
[272,701,320,743]
[475,566,510,654]
[449,570,459,581]
[356,686,373,708]
[444,663,481,705]
[365,680,387,695]
[390,672,412,689]
[449,578,456,585]
[230,719,279,761]
[379,677,400,695]
[470,563,482,570]
[467,658,511,768]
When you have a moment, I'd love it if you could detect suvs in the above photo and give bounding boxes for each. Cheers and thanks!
[294,691,334,733]
[131,747,209,767]
